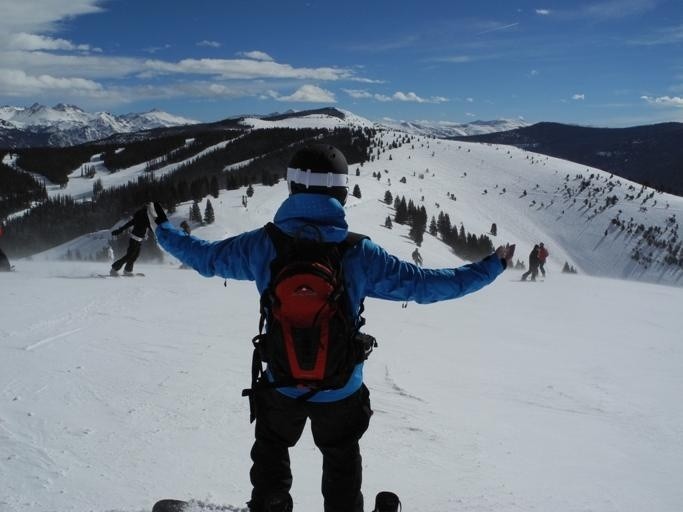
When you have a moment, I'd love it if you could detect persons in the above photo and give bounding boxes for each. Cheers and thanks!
[109,203,150,276]
[146,144,517,512]
[521,244,540,280]
[411,247,422,267]
[539,242,549,276]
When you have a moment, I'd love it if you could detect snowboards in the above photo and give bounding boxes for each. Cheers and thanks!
[90,273,144,279]
[153,498,252,512]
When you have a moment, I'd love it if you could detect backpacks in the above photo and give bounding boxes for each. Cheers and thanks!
[262,221,372,390]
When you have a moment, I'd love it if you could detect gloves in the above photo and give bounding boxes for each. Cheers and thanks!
[146,200,168,236]
[488,243,517,270]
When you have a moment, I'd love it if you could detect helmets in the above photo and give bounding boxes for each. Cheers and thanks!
[285,142,350,209]
